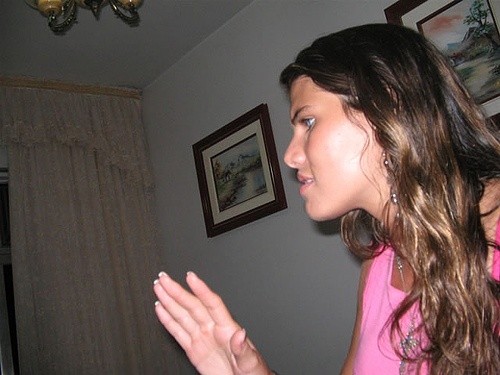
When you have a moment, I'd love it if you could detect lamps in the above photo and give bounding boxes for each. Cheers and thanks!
[24,0,143,34]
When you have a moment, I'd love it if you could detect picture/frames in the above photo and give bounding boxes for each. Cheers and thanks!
[383,0,500,134]
[191,102,289,240]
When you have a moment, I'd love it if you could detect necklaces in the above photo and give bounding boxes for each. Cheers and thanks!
[385,225,432,375]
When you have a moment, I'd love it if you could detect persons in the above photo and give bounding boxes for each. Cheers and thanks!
[152,24,499,375]
